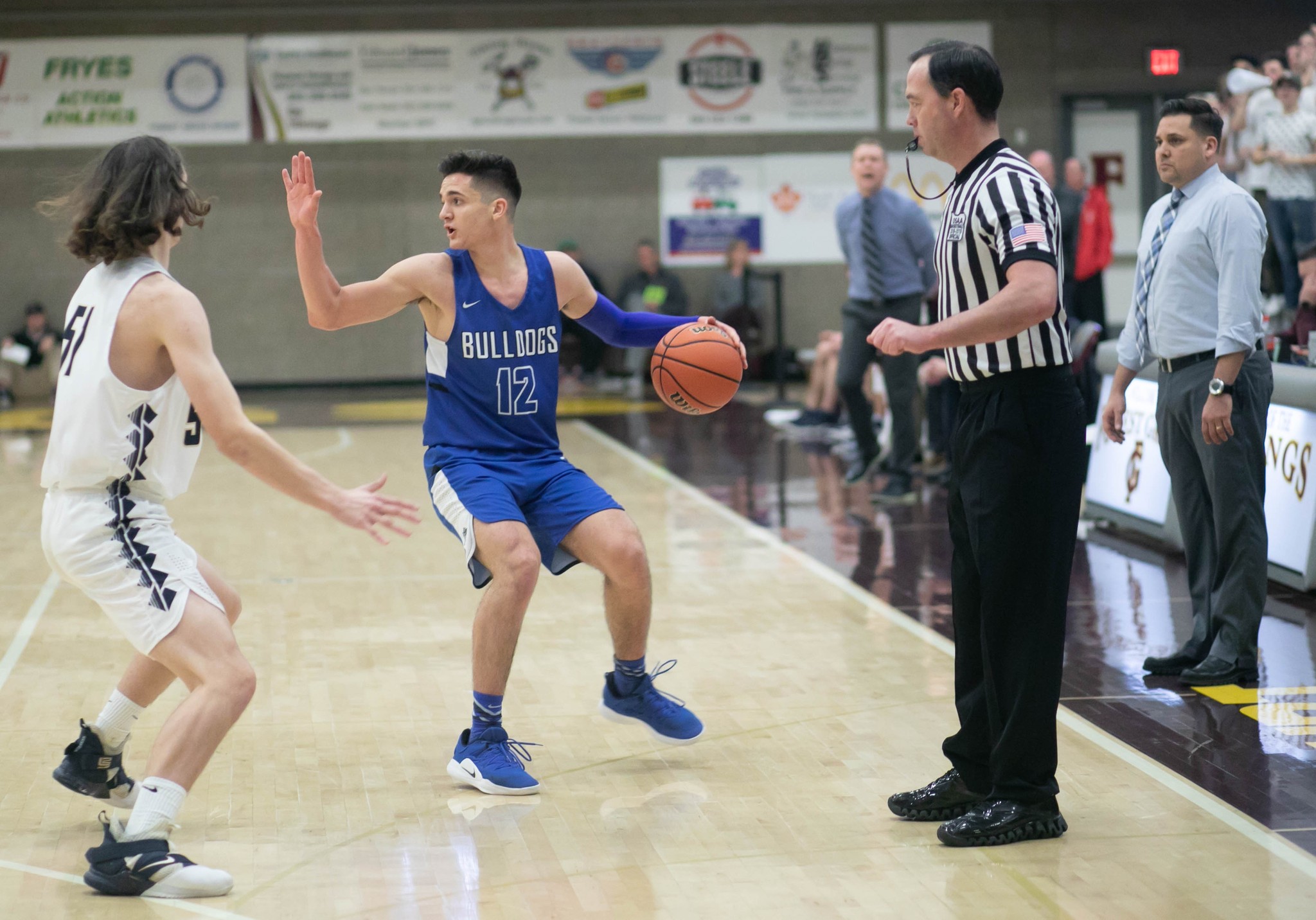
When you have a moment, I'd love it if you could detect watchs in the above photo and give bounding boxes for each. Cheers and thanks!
[1208,378,1233,395]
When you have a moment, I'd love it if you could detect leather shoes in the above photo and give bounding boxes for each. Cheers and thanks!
[1143,652,1203,674]
[1180,655,1260,684]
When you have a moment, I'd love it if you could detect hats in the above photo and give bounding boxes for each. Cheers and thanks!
[1276,75,1302,92]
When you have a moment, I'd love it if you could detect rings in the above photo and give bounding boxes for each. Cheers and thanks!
[1216,426,1223,430]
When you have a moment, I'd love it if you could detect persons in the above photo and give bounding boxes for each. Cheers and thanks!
[0,303,63,410]
[39,136,422,897]
[544,22,1316,508]
[864,39,1085,843]
[283,151,747,795]
[1102,98,1273,688]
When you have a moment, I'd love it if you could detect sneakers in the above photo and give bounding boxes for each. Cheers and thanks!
[53,718,142,810]
[937,795,1068,846]
[599,671,705,745]
[888,767,988,820]
[446,727,543,795]
[84,810,233,899]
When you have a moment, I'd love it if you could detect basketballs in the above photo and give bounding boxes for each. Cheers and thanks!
[649,321,743,417]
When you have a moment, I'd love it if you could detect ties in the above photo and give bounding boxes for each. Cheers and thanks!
[1134,190,1185,367]
[861,199,883,318]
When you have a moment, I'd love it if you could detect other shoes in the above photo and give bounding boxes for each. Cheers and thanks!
[867,476,917,503]
[844,444,881,481]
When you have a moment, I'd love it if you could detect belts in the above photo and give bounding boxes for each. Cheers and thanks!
[850,293,921,307]
[1159,337,1263,372]
